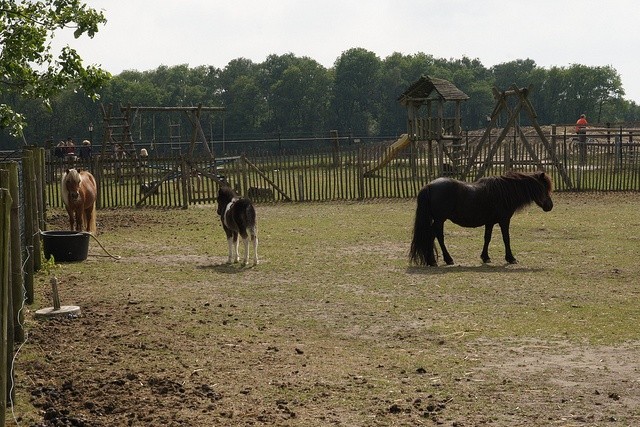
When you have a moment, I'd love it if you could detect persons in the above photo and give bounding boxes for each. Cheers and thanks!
[575,113,590,135]
[55,141,64,158]
[64,137,76,153]
[80,140,92,160]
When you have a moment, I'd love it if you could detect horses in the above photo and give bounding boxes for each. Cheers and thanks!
[61,168,98,235]
[216,186,260,266]
[408,171,554,267]
